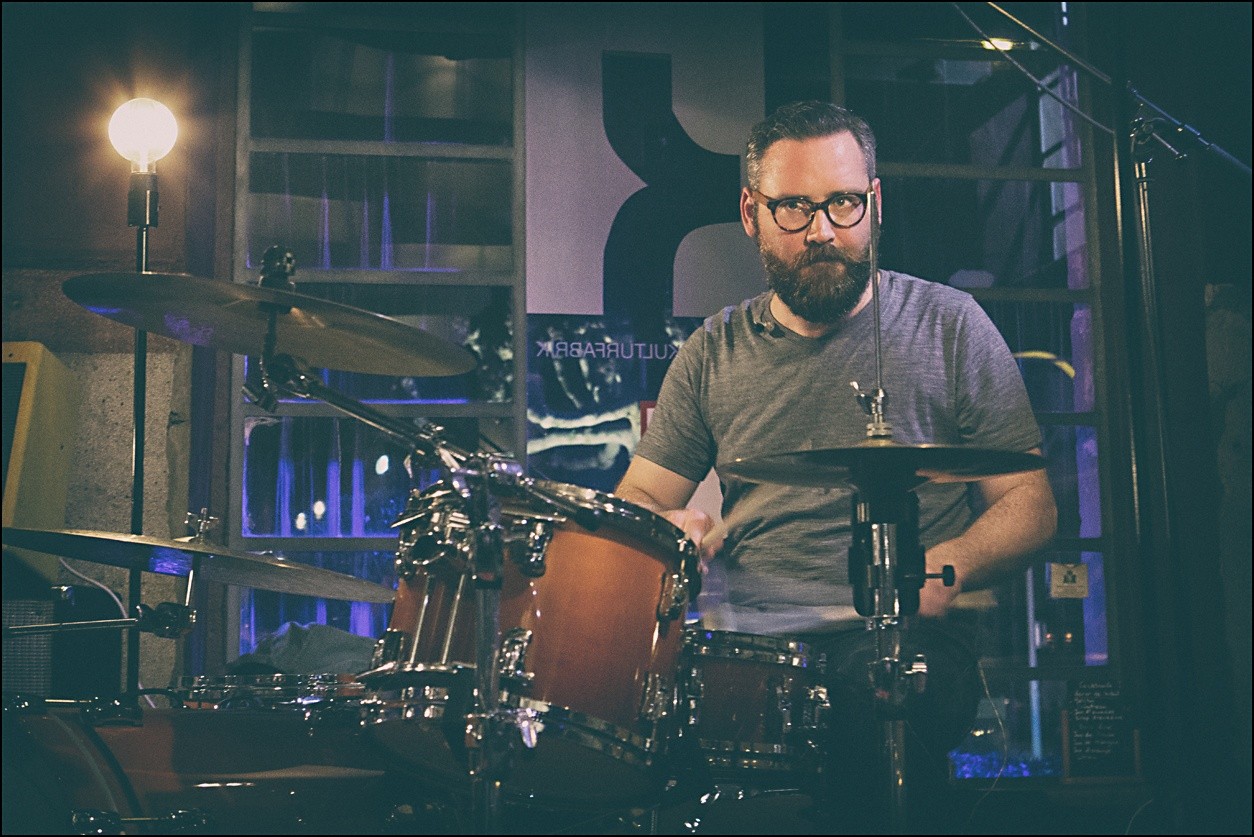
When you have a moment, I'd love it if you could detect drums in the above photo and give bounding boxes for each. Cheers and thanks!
[162,676,381,835]
[670,622,827,788]
[371,468,701,778]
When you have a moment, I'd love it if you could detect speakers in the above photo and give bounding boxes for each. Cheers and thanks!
[1,585,122,701]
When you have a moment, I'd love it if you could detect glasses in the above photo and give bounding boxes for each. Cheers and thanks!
[749,185,872,233]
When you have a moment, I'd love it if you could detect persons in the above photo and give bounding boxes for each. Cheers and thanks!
[615,103,1058,750]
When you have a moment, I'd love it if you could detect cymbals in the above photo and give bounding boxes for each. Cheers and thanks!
[1,525,399,606]
[713,438,1050,496]
[61,269,477,378]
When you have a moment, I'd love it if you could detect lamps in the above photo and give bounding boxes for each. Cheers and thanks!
[108,97,179,713]
